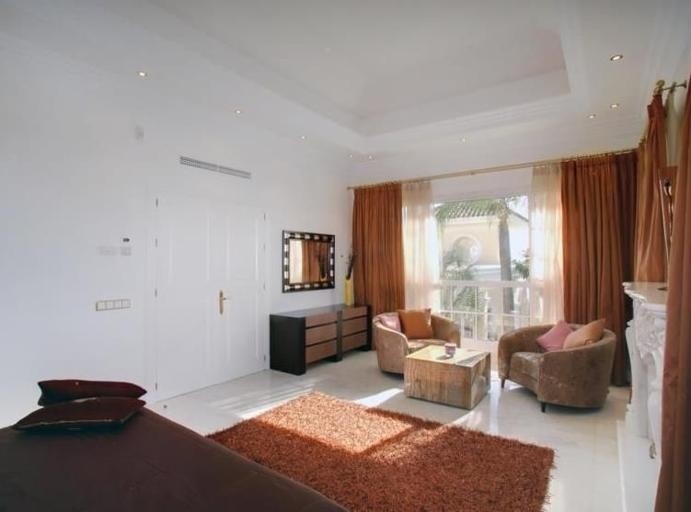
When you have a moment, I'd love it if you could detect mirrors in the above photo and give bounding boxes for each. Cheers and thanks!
[282,230,335,293]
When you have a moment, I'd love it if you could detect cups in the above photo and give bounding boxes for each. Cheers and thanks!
[445,343,456,358]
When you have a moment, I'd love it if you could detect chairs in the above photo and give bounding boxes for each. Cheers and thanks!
[498,325,616,412]
[372,312,461,374]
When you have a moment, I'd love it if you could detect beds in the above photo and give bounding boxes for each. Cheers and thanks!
[0,404,347,512]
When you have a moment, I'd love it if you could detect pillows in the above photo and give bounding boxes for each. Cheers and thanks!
[37,380,146,404]
[536,320,572,351]
[399,309,433,339]
[11,397,145,430]
[564,318,604,349]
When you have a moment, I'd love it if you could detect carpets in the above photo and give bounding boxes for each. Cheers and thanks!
[206,392,554,512]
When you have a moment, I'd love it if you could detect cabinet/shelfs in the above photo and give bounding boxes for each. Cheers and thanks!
[270,303,370,373]
[621,281,669,512]
